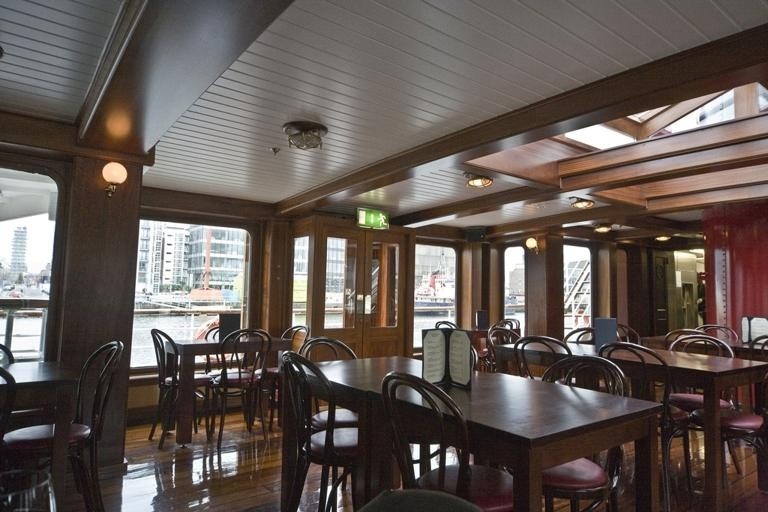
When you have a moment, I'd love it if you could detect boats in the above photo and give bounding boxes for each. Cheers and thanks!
[413,263,455,315]
[504,295,518,315]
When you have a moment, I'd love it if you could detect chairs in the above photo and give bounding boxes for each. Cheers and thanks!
[0,339,125,511]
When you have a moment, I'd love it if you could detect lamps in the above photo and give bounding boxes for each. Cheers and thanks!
[102,162,128,198]
[282,121,328,149]
[525,237,540,256]
[463,172,494,190]
[593,224,612,233]
[569,196,595,209]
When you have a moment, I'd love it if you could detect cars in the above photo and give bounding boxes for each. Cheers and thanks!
[3,283,24,300]
